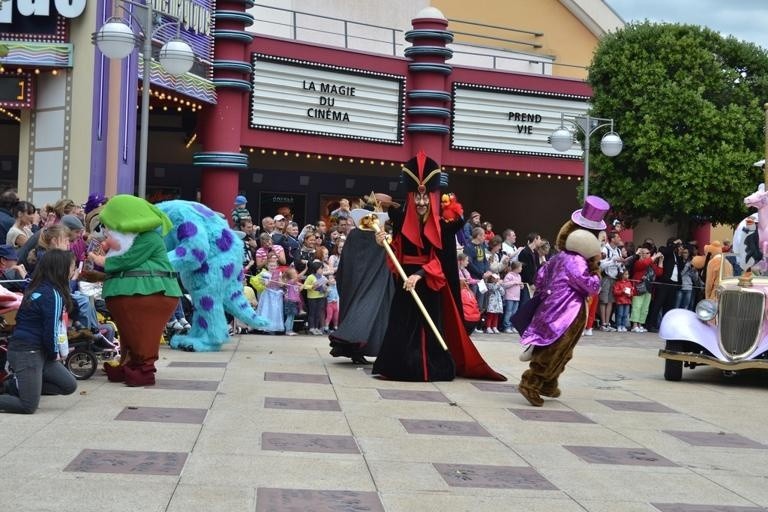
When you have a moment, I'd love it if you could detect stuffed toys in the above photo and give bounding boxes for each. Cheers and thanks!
[520,195,610,407]
[154,200,269,352]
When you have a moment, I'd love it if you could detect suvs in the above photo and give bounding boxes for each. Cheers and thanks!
[656,180,768,382]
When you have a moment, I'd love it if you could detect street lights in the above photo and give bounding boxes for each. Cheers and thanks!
[548,110,625,207]
[86,1,199,202]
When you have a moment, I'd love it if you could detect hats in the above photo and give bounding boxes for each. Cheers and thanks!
[0,245,19,260]
[233,196,247,205]
[60,215,83,230]
[274,214,286,221]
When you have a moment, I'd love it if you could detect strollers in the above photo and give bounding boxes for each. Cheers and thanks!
[0,284,120,382]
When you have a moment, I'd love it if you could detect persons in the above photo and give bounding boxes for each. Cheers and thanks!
[89,194,183,387]
[0,189,120,415]
[228,196,357,336]
[167,301,191,329]
[455,211,550,334]
[329,193,400,365]
[372,182,507,382]
[580,219,732,336]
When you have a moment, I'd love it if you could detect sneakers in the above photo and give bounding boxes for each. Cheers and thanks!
[600,323,648,332]
[585,329,592,336]
[486,327,518,334]
[470,329,483,335]
[166,319,191,329]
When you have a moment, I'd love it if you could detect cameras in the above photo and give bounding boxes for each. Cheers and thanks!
[268,246,273,252]
